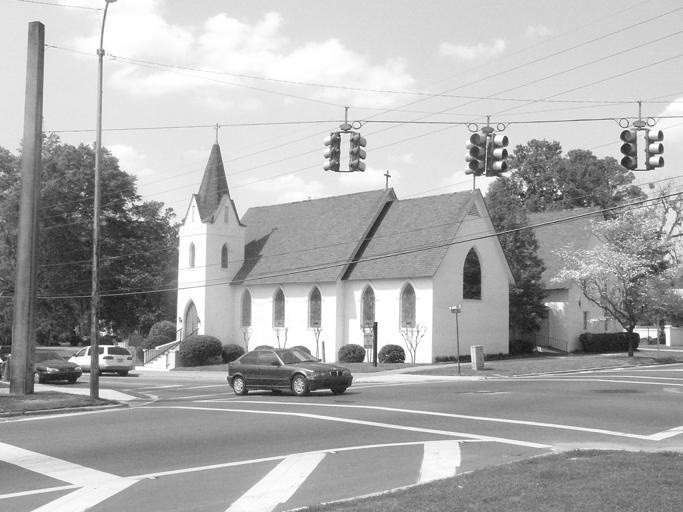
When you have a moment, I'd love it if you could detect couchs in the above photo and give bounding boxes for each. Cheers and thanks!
[349,132,367,173]
[465,132,510,177]
[645,128,665,173]
[619,128,638,171]
[323,131,342,172]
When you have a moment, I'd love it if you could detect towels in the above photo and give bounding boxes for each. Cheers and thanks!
[471,345,484,370]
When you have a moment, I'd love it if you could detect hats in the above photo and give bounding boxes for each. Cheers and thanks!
[3,349,83,383]
[227,347,353,397]
[67,345,132,376]
